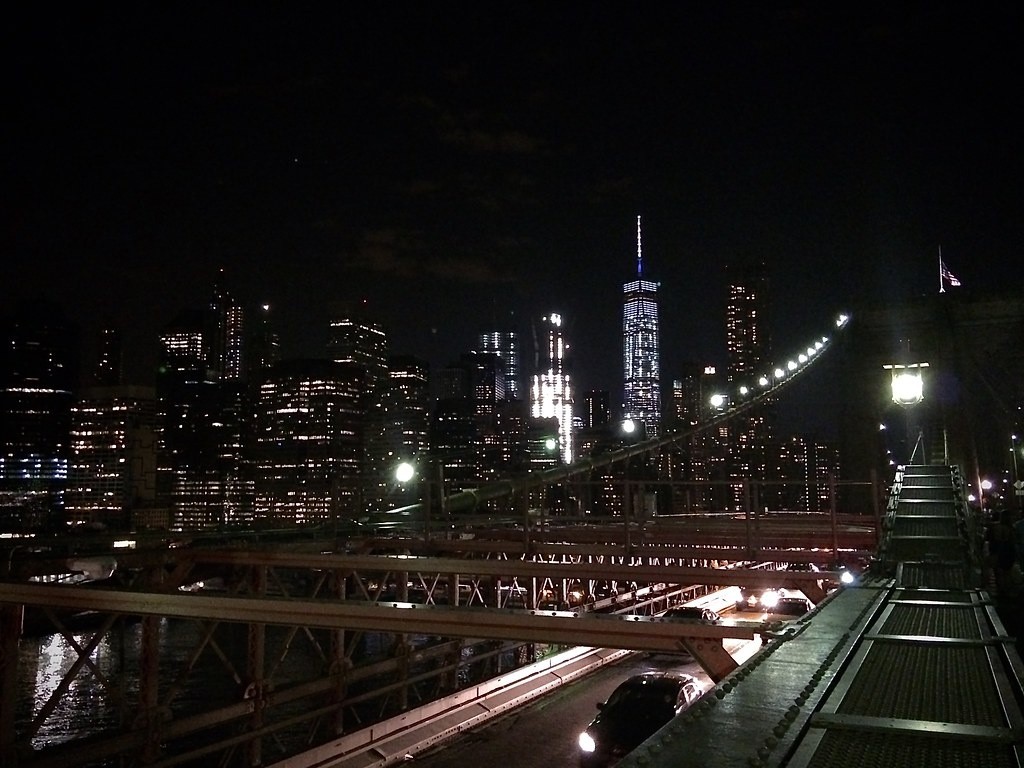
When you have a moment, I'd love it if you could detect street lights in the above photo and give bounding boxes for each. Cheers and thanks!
[882,337,929,465]
[1010,435,1018,480]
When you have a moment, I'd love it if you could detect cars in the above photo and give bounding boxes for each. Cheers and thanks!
[643,607,720,657]
[579,672,705,758]
[736,586,781,611]
[821,559,853,590]
[759,598,811,644]
